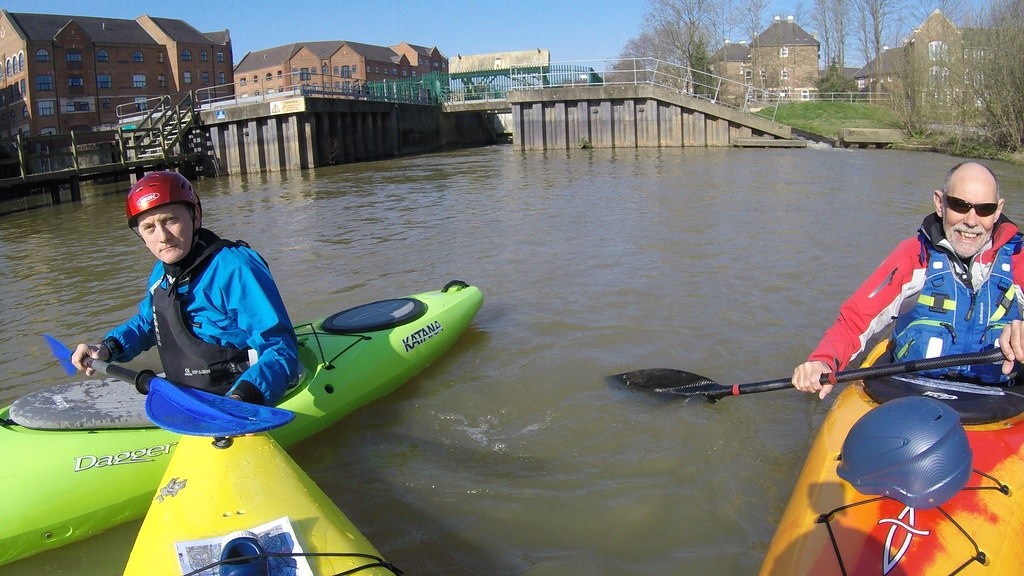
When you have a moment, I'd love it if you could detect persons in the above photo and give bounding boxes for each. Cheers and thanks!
[790,161,1024,400]
[70,171,298,406]
[353,79,371,99]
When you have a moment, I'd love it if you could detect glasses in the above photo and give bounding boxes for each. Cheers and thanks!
[946,195,998,217]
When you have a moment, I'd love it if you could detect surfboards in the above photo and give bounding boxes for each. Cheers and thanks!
[603,349,1024,413]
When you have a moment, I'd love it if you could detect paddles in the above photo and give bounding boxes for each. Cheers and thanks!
[32,331,296,444]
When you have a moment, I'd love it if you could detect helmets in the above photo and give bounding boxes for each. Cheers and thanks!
[125,171,199,228]
[836,396,973,509]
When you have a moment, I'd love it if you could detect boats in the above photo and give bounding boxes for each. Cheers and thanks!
[756,335,1024,576]
[0,281,482,575]
